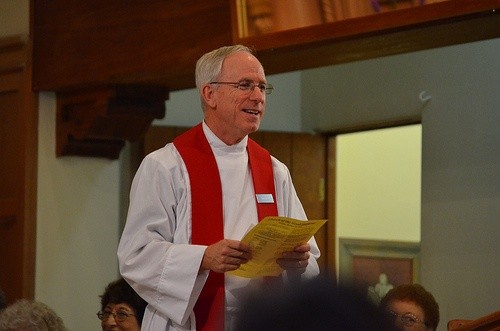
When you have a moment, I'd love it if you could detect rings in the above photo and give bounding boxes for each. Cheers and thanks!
[299,261,302,266]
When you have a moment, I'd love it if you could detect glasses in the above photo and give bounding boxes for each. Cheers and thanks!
[389,311,426,326]
[208,79,274,94]
[97,309,137,321]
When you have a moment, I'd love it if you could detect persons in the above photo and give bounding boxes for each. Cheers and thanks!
[0,301,68,331]
[96,278,148,331]
[117,45,320,331]
[224,274,439,331]
